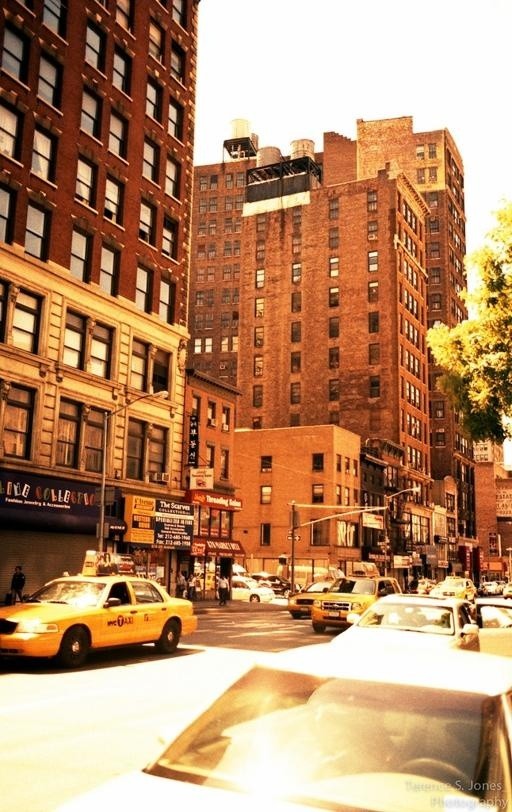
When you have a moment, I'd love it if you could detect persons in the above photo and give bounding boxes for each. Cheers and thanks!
[97,553,118,574]
[216,574,230,606]
[467,604,512,628]
[175,570,187,597]
[187,572,197,601]
[11,565,26,605]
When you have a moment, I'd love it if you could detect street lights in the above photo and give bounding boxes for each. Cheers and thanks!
[505,547,512,578]
[384,487,422,579]
[96,390,171,549]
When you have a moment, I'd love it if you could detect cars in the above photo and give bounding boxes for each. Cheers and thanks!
[225,569,512,660]
[0,549,201,669]
[53,636,512,811]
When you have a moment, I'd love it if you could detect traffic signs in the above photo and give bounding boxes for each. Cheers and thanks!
[286,535,301,540]
